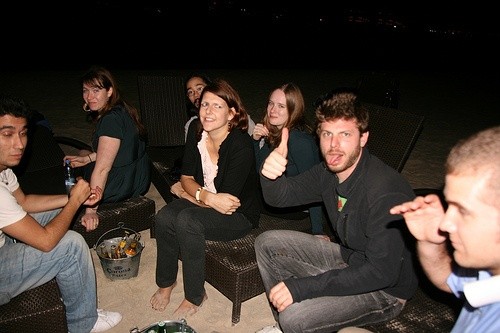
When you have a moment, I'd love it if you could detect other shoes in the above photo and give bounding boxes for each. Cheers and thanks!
[256,325,283,333]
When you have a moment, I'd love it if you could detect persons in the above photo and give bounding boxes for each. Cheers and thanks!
[255,94,422,333]
[184,73,212,133]
[339,127,500,333]
[0,91,122,333]
[63,67,150,232]
[150,79,260,320]
[249,82,330,241]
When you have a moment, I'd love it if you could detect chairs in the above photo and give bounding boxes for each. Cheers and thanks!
[0,73,478,333]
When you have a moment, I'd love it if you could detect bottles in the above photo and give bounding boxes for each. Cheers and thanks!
[101,230,141,259]
[64,160,75,193]
[147,318,187,333]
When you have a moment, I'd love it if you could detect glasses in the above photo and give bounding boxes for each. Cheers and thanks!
[186,86,206,97]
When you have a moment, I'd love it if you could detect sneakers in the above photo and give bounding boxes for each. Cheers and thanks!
[90,309,123,333]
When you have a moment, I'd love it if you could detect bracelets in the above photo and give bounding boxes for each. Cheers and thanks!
[88,154,92,162]
[196,187,206,203]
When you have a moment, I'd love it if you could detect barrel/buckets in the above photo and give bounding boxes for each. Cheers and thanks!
[94,221,146,280]
[130,317,196,333]
